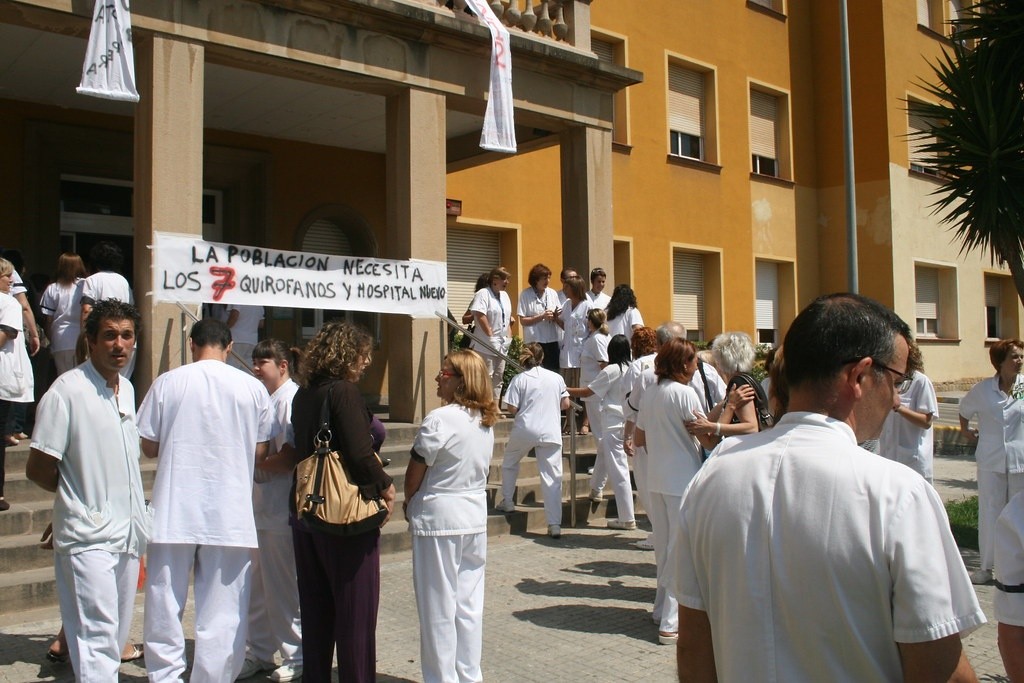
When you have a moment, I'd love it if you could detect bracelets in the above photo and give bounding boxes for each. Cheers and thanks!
[895,404,901,412]
[714,421,721,437]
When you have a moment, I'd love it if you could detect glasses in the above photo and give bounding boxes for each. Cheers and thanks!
[841,356,914,395]
[438,370,458,378]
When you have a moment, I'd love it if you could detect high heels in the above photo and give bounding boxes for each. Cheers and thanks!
[565,424,572,435]
[580,423,590,435]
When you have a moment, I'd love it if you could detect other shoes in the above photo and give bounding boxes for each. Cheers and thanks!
[3,434,19,446]
[0,496,10,510]
[12,432,29,439]
[970,569,993,583]
[636,540,654,550]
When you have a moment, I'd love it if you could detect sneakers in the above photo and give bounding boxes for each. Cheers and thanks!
[608,520,637,529]
[589,488,603,502]
[496,499,515,512]
[548,525,561,538]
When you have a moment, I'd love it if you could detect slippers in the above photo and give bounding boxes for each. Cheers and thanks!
[654,618,679,645]
[46,648,70,662]
[121,643,144,663]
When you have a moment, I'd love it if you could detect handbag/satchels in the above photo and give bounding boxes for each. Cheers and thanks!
[736,372,776,432]
[459,324,476,348]
[288,379,390,540]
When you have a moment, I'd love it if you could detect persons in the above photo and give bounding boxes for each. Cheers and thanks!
[0,242,396,683]
[657,292,987,683]
[992,491,1024,683]
[402,349,500,683]
[456,264,940,642]
[955,338,1023,579]
